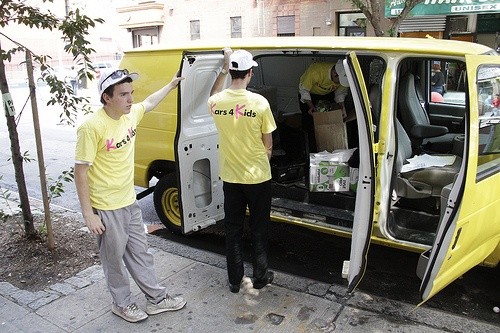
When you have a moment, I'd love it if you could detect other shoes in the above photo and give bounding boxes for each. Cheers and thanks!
[229,282,240,293]
[253,272,274,289]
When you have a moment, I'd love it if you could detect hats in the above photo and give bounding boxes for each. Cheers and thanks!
[98,68,139,95]
[229,50,258,71]
[334,59,350,88]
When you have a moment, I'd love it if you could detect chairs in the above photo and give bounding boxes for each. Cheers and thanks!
[393,117,462,199]
[399,73,465,153]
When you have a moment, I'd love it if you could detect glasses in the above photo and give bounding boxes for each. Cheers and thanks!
[100,69,129,90]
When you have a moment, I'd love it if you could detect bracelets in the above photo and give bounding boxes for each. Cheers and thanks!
[221,69,228,74]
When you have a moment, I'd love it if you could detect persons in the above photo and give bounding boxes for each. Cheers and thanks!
[208,48,277,292]
[74,68,187,322]
[69,66,76,91]
[432,64,444,93]
[299,60,350,152]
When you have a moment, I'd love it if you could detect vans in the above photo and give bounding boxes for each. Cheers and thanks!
[111,37,500,314]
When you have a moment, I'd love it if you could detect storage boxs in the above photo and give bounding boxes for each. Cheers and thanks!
[312,109,356,151]
[309,148,357,192]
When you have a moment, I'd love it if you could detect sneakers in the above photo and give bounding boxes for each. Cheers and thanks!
[112,302,148,322]
[145,293,187,315]
